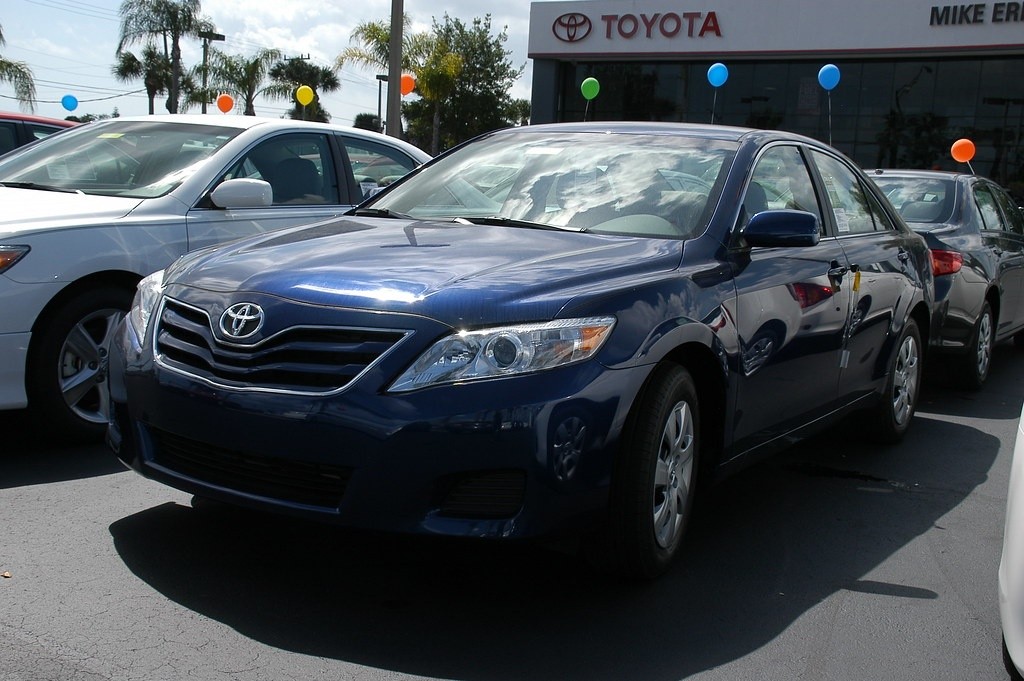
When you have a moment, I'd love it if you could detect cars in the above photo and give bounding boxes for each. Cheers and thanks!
[0,110,415,190]
[655,170,713,193]
[768,169,1024,393]
[103,117,935,583]
[0,111,505,445]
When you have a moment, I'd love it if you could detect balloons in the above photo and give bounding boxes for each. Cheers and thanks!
[818,64,840,90]
[62,95,78,112]
[401,74,415,95]
[951,139,975,162]
[707,63,728,87]
[296,85,314,105]
[217,94,233,112]
[581,78,600,99]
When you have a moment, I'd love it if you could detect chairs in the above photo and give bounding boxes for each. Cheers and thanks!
[745,182,769,215]
[267,158,321,202]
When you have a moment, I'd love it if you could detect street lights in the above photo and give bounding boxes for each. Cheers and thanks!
[196,28,225,113]
[376,74,388,134]
